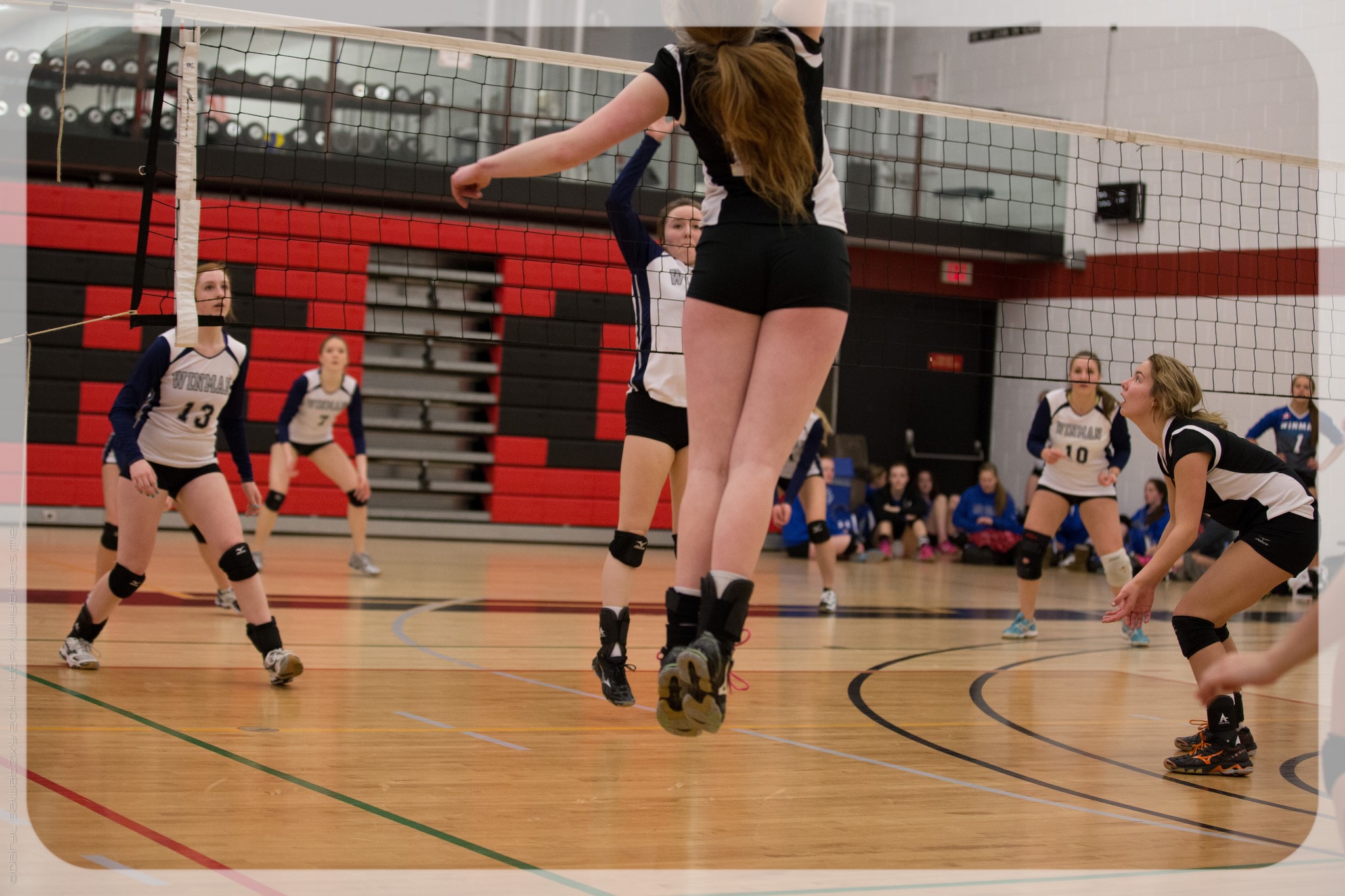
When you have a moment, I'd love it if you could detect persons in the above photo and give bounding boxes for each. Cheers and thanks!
[592,116,708,706]
[1001,350,1151,646]
[249,334,381,579]
[449,0,853,735]
[1194,563,1344,810]
[756,392,1242,619]
[1101,354,1316,776]
[59,263,301,687]
[1247,371,1345,603]
[94,425,246,616]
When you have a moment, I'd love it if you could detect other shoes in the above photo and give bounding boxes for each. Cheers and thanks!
[1263,582,1317,600]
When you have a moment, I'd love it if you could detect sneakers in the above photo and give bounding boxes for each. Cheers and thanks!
[591,656,637,707]
[1119,620,1150,646]
[917,544,934,562]
[657,646,703,737]
[348,549,381,575]
[1002,611,1038,638]
[818,590,837,613]
[215,587,243,615]
[676,627,751,734]
[262,648,304,685]
[1164,732,1254,777]
[1174,720,1257,756]
[58,636,101,669]
[879,541,893,561]
[936,540,958,555]
[250,551,263,569]
